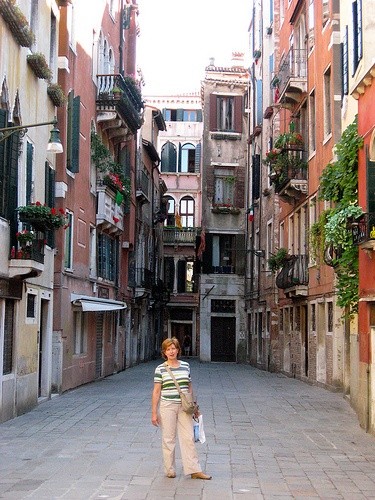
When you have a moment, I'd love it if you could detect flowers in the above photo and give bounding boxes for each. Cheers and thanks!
[15,201,65,261]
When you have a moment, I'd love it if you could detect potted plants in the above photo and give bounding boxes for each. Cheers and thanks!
[211,203,241,216]
[90,72,145,206]
[262,131,310,194]
[266,246,289,271]
[0,0,67,108]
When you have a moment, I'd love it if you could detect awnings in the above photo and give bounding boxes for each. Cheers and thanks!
[70,294,128,312]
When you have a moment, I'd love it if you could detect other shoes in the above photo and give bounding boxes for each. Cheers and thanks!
[191,472,212,480]
[167,474,175,478]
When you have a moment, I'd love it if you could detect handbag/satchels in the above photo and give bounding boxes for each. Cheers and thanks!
[193,415,207,444]
[180,392,195,414]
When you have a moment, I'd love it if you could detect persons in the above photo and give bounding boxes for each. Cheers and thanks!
[152,336,212,480]
